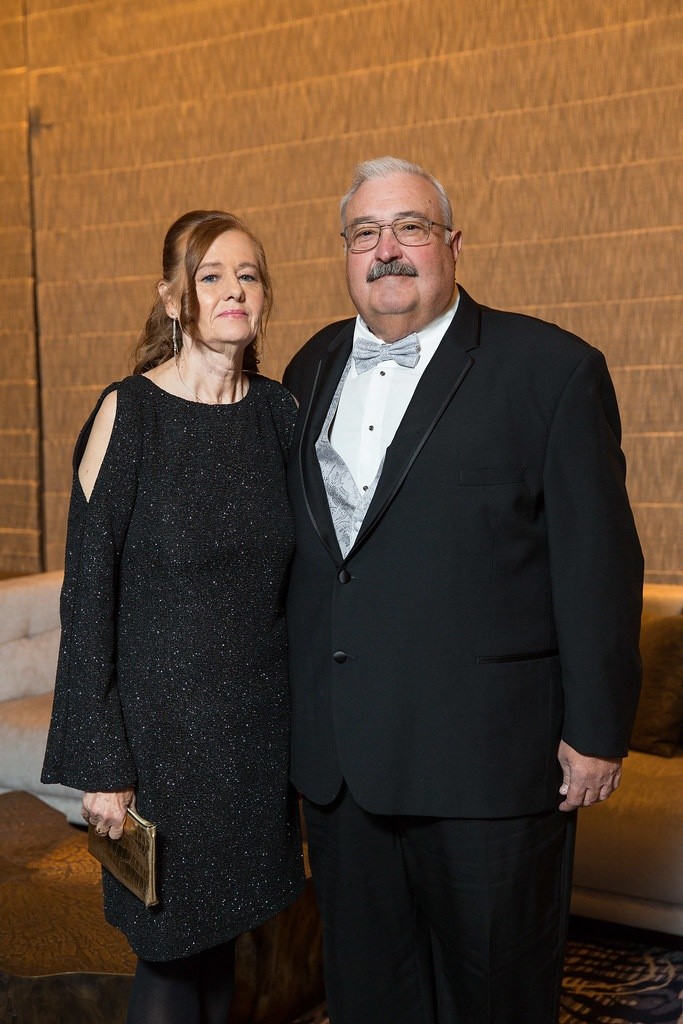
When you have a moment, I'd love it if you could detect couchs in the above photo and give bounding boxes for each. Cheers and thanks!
[570,583,683,938]
[0,569,84,827]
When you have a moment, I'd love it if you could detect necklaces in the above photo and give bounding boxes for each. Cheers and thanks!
[174,360,205,403]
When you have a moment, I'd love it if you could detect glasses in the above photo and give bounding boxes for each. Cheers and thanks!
[340,216,452,253]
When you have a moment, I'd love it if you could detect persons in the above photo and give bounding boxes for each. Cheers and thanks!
[281,156,645,1024]
[37,212,317,1024]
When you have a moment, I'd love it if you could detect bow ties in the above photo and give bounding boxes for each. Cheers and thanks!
[352,332,422,375]
